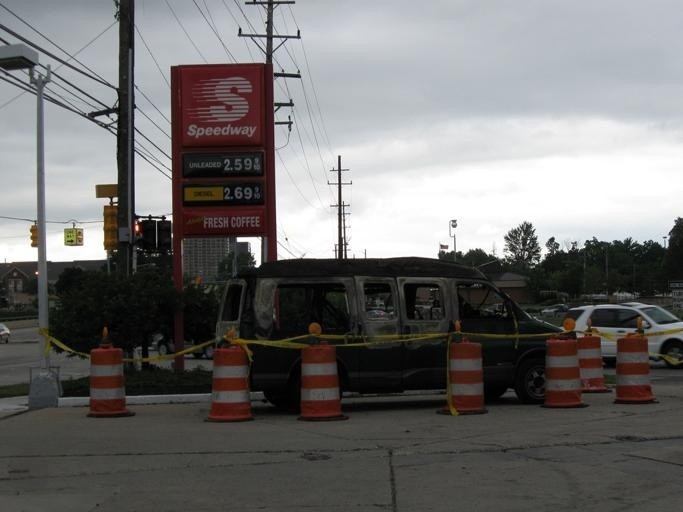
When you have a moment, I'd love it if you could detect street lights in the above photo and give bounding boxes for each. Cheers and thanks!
[448,219,458,263]
[0,41,52,366]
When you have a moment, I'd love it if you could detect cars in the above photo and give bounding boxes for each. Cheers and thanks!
[155,331,215,360]
[541,303,568,318]
[0,324,10,344]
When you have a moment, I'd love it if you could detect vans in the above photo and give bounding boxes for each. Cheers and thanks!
[216,256,570,414]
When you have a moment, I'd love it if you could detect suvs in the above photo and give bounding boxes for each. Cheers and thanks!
[559,301,683,370]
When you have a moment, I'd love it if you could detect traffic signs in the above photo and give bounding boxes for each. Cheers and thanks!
[64,228,77,245]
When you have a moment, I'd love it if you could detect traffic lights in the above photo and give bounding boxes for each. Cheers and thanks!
[30,225,38,248]
